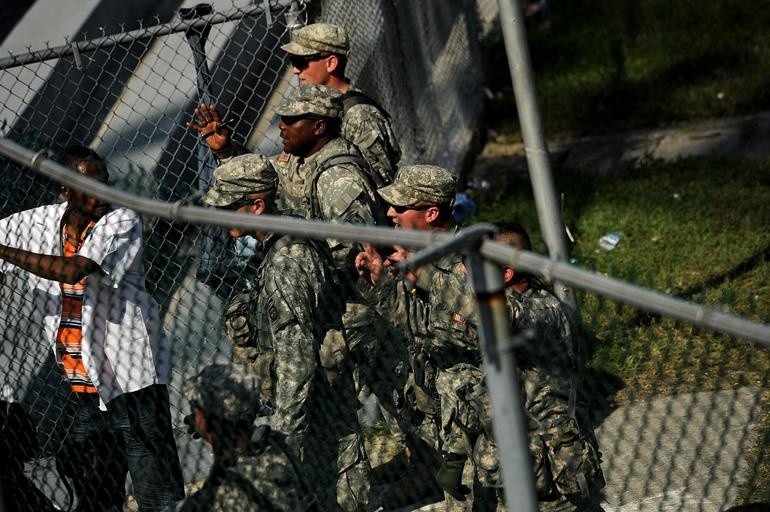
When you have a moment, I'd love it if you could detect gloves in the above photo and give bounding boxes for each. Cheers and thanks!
[437,454,470,501]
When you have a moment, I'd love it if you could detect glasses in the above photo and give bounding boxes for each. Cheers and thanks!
[289,54,323,64]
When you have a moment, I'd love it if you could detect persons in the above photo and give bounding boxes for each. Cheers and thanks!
[175,21,603,512]
[0,145,185,512]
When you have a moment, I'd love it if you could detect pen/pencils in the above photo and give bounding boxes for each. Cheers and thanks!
[201,119,234,137]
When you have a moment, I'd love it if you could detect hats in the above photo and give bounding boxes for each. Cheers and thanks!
[183,364,261,421]
[200,153,279,207]
[273,84,344,118]
[280,23,349,56]
[376,165,459,207]
